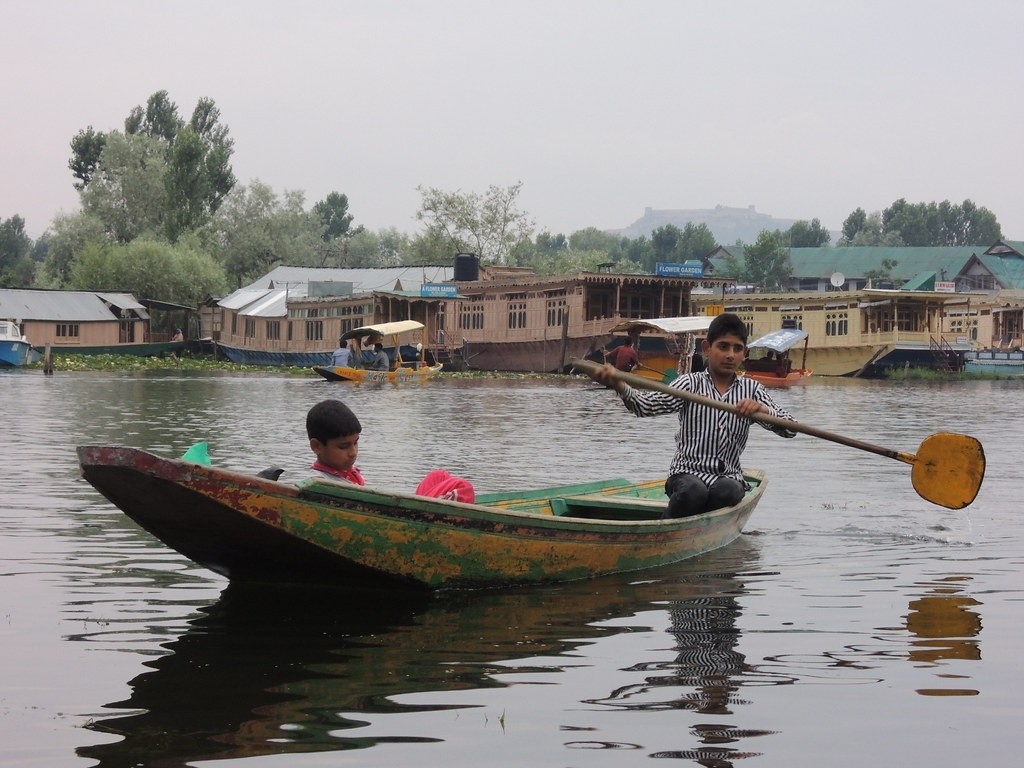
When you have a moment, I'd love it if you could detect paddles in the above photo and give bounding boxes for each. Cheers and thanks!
[569,360,987,510]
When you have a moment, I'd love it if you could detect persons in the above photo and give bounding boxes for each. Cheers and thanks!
[331,340,355,367]
[759,352,787,371]
[590,314,798,519]
[370,343,390,371]
[306,400,475,504]
[169,329,183,341]
[604,337,642,373]
[688,348,704,373]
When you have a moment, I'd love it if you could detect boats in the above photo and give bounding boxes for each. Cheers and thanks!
[30,337,212,359]
[211,337,427,367]
[313,320,442,383]
[76,445,769,593]
[0,321,43,367]
[603,319,816,391]
